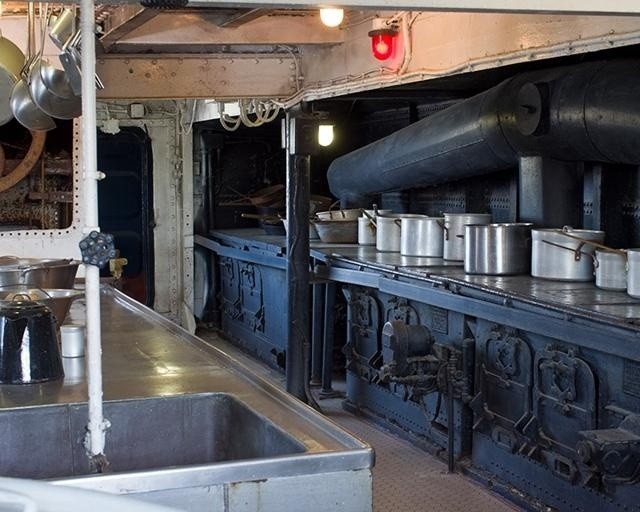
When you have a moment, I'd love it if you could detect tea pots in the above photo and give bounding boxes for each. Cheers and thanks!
[0,291,65,387]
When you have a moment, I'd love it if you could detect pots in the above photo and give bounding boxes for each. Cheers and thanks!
[541,229,640,299]
[357,216,377,245]
[375,212,429,253]
[0,2,106,134]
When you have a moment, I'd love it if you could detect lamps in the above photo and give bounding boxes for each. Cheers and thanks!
[368,18,398,62]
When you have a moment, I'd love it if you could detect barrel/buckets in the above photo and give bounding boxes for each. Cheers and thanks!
[372,216,429,252]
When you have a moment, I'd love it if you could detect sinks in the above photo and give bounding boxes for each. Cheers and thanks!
[0,388,314,487]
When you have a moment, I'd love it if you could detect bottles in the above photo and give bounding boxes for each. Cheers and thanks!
[59,324,84,358]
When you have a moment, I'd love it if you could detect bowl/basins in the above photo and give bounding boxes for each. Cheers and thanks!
[0,258,86,327]
[278,194,363,244]
